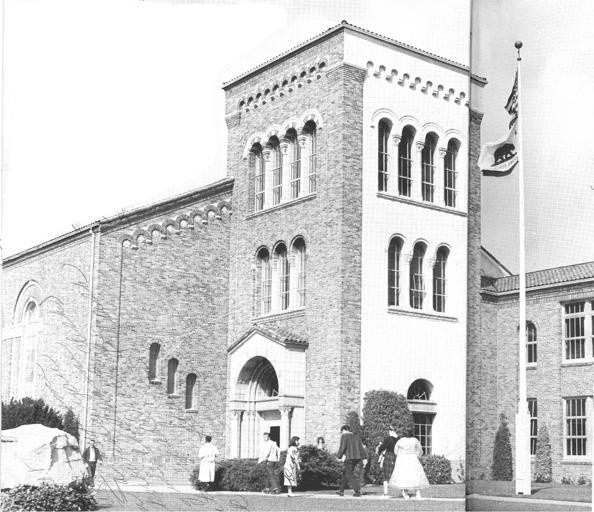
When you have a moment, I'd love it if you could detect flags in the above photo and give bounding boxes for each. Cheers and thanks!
[477,67,518,177]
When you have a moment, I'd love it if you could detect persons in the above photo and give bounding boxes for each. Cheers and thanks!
[359,441,377,486]
[197,436,220,492]
[388,427,429,499]
[258,432,279,494]
[378,425,406,497]
[317,437,328,453]
[335,424,360,497]
[82,440,103,487]
[283,436,302,497]
